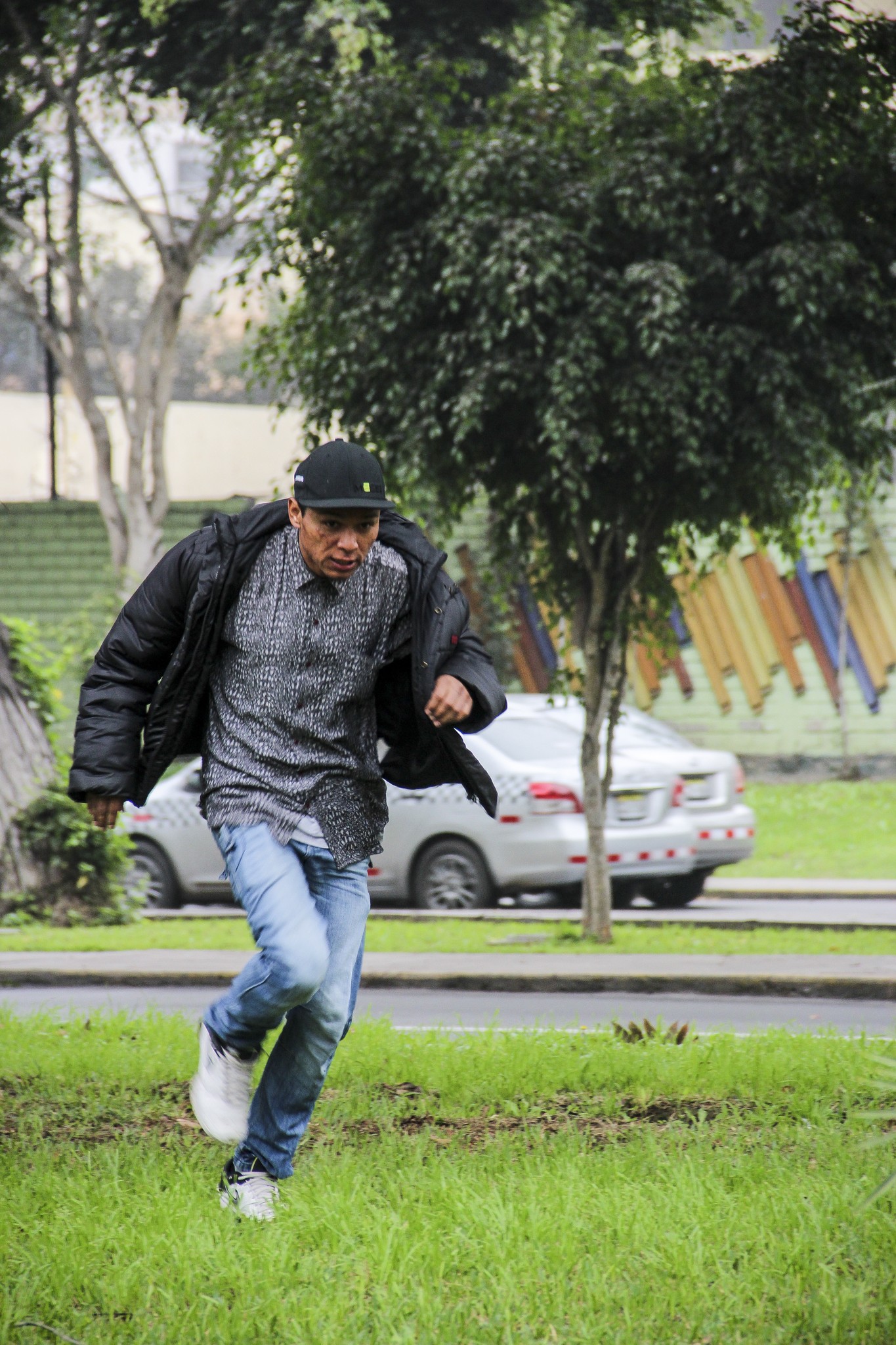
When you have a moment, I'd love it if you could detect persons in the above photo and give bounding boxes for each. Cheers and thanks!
[70,436,510,1226]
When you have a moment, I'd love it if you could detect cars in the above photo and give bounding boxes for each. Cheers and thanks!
[114,693,759,912]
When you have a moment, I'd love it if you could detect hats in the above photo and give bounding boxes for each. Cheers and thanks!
[295,440,394,509]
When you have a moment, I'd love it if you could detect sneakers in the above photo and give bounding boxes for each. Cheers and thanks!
[218,1157,280,1220]
[190,1021,261,1142]
